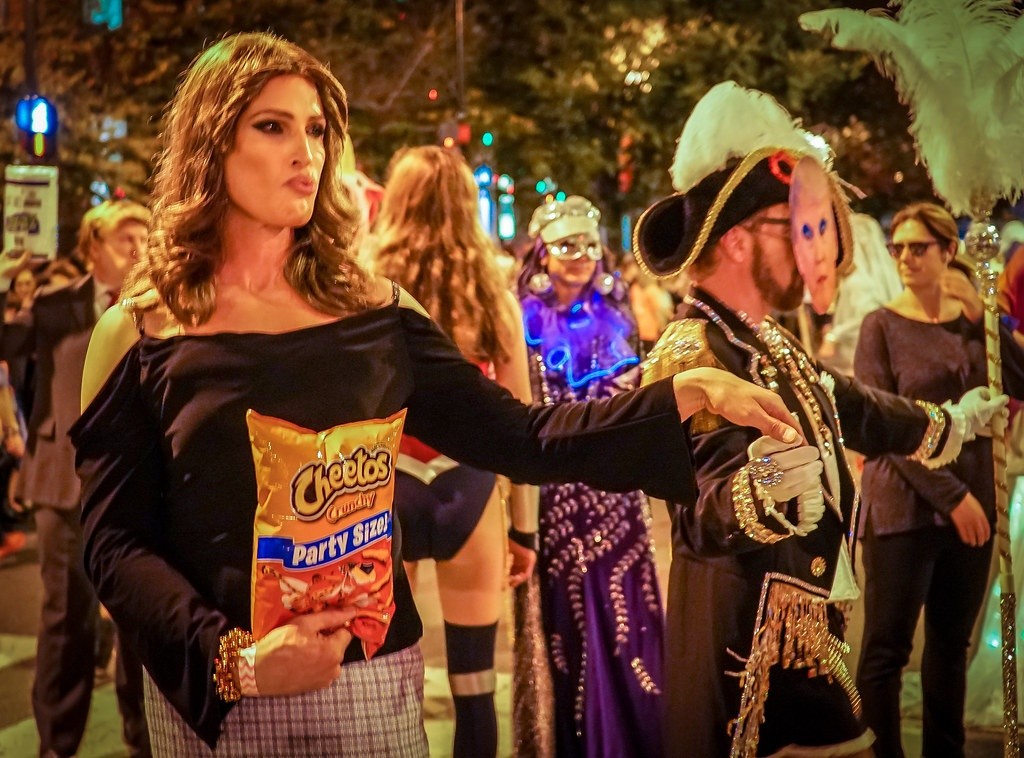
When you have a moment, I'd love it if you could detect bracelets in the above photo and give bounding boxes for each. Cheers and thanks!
[213,627,255,702]
[508,527,539,551]
[753,477,826,536]
[904,399,946,460]
[732,459,795,544]
[239,643,260,699]
[749,456,783,487]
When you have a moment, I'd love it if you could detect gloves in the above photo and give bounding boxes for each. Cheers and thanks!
[747,433,822,502]
[960,386,1010,438]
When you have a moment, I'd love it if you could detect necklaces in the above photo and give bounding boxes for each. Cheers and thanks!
[739,312,833,456]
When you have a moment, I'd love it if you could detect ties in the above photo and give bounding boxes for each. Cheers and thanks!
[107,290,121,311]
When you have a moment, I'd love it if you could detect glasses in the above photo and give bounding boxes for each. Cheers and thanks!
[886,242,939,259]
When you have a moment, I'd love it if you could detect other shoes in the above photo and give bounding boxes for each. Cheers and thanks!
[0,532,25,559]
[93,674,113,688]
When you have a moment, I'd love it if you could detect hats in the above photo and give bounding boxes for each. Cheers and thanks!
[529,195,602,243]
[632,82,853,280]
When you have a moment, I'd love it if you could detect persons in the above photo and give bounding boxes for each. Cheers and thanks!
[821,181,1024,758]
[1,195,676,758]
[365,146,540,758]
[623,79,1010,758]
[68,31,809,758]
